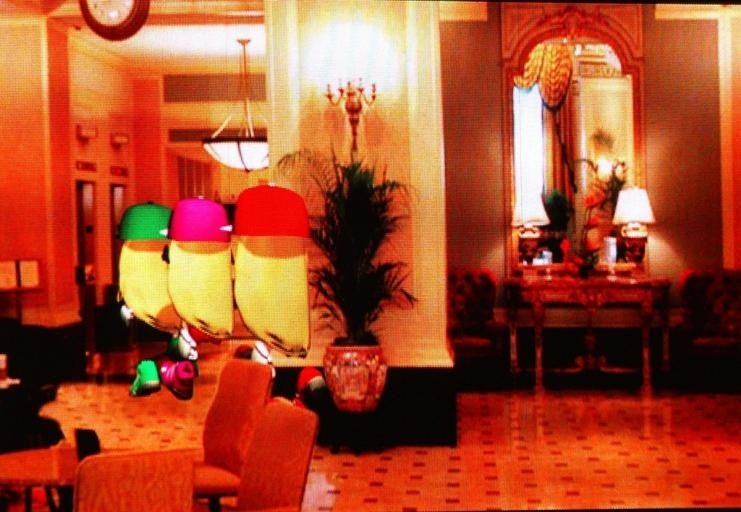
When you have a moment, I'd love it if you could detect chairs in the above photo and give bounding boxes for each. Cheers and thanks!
[664,269,740,394]
[72,356,320,510]
[447,269,509,386]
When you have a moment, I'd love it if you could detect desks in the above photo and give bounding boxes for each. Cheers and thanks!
[504,275,672,411]
[1,447,132,511]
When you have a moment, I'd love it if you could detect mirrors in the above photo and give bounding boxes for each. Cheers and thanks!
[500,1,649,279]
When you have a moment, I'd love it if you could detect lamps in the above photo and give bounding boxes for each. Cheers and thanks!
[324,76,385,153]
[199,35,272,174]
[611,187,656,265]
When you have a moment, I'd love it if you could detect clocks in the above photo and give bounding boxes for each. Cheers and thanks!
[79,0,150,42]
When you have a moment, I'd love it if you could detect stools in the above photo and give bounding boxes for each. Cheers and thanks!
[319,409,395,457]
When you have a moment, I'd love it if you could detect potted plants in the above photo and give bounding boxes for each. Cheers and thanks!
[310,162,411,414]
